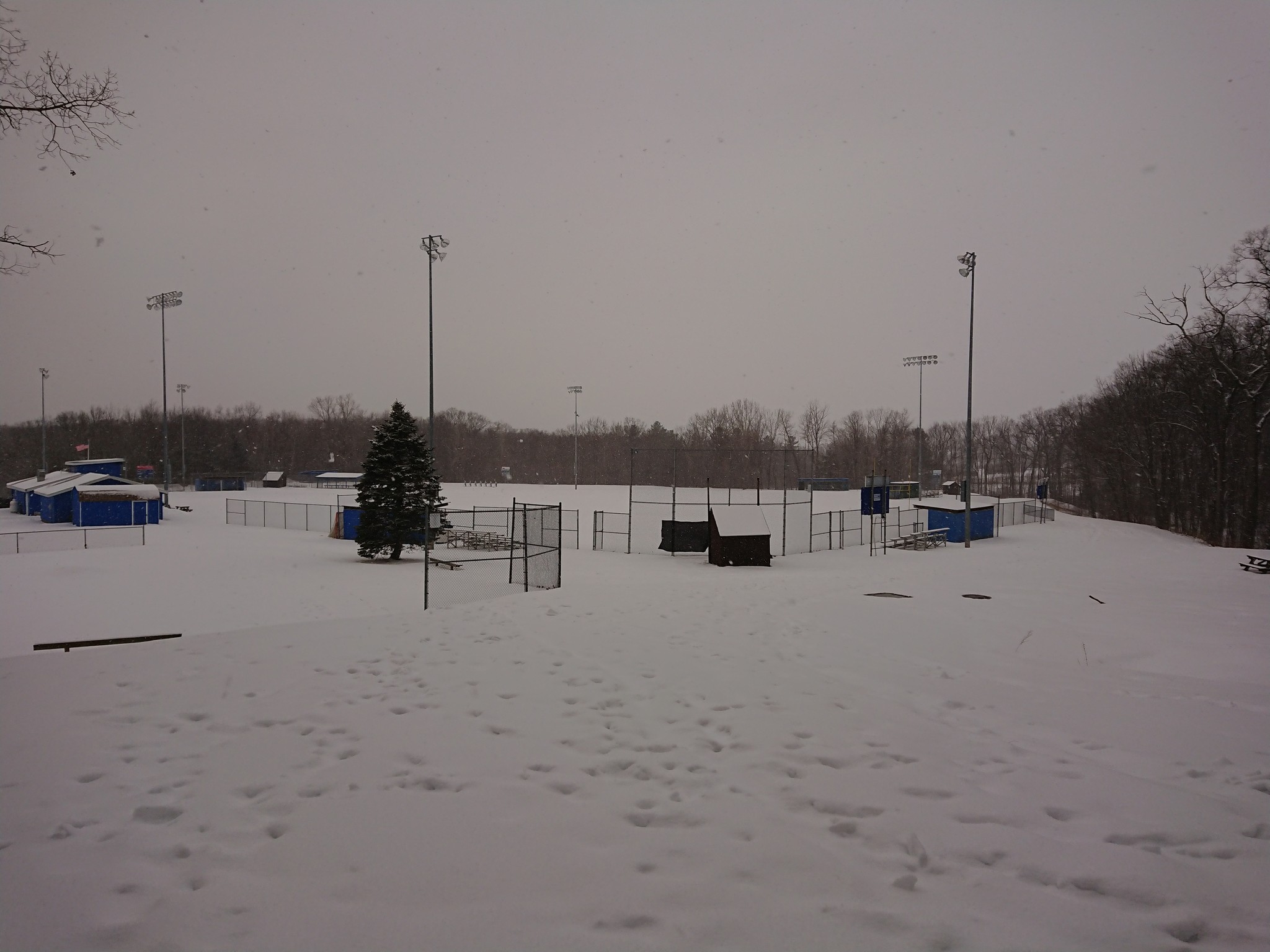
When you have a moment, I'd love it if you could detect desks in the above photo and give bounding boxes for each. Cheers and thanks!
[175,506,191,512]
[1247,555,1270,568]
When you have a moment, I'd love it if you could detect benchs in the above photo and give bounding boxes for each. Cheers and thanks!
[324,485,356,489]
[33,632,182,652]
[429,557,463,571]
[1239,563,1270,575]
[444,528,519,552]
[185,508,193,512]
[881,527,950,551]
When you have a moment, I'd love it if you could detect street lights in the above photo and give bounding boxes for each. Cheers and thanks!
[902,354,938,501]
[958,251,977,549]
[146,290,183,508]
[176,384,191,491]
[419,234,450,478]
[566,386,582,490]
[39,368,49,470]
